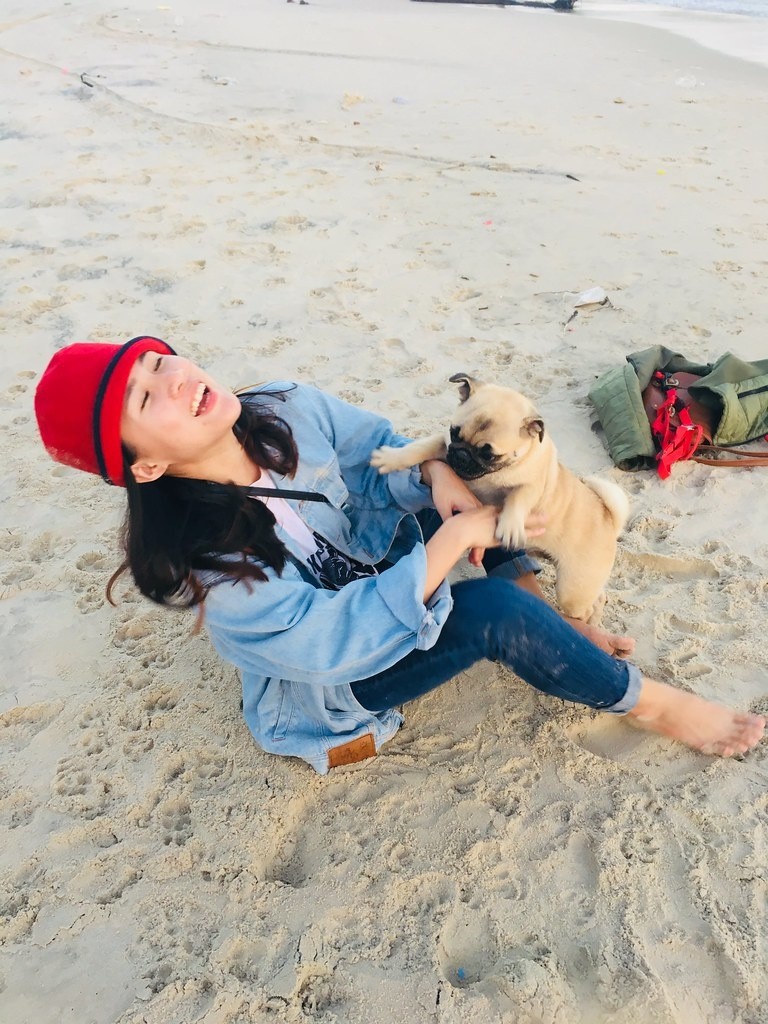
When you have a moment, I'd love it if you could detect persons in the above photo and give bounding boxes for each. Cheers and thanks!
[33,336,766,773]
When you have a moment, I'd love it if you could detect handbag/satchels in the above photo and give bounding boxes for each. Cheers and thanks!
[643,369,768,480]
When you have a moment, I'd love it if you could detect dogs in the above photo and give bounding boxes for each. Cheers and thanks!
[371,373,632,628]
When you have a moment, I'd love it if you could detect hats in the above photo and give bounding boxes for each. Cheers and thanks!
[35,336,177,488]
